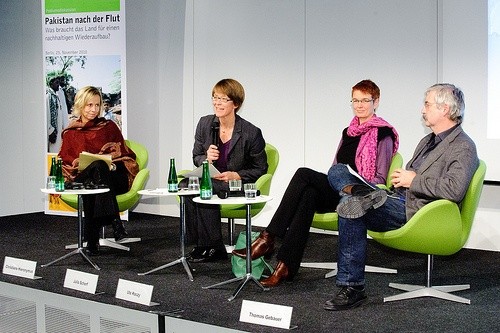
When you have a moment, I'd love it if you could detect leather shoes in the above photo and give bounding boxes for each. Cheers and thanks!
[189,246,228,264]
[85,236,100,255]
[336,189,387,219]
[178,245,203,260]
[323,286,365,310]
[113,220,128,242]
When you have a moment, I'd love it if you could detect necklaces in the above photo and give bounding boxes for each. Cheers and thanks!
[222,130,225,133]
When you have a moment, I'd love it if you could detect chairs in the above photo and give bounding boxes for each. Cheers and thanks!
[58,142,149,253]
[367,158,486,304]
[174,142,280,253]
[300,154,403,279]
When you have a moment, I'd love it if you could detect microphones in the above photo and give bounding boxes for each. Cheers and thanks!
[211,121,220,149]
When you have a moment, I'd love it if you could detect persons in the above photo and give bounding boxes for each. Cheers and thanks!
[232,79,399,287]
[177,78,269,263]
[322,83,480,310]
[57,86,139,255]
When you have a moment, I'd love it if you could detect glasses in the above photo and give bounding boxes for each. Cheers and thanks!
[351,98,375,104]
[212,96,232,103]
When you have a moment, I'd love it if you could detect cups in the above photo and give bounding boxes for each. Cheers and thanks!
[188,177,200,191]
[229,180,242,191]
[244,183,256,199]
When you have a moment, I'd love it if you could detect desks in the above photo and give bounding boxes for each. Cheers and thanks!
[135,185,212,282]
[37,188,111,271]
[192,192,273,301]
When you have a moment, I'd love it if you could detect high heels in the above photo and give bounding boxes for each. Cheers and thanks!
[232,230,274,262]
[260,261,293,286]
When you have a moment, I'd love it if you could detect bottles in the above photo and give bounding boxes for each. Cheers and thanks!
[47,156,57,189]
[55,158,65,191]
[167,158,179,192]
[199,160,212,200]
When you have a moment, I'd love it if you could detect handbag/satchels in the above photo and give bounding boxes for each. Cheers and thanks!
[230,231,274,280]
